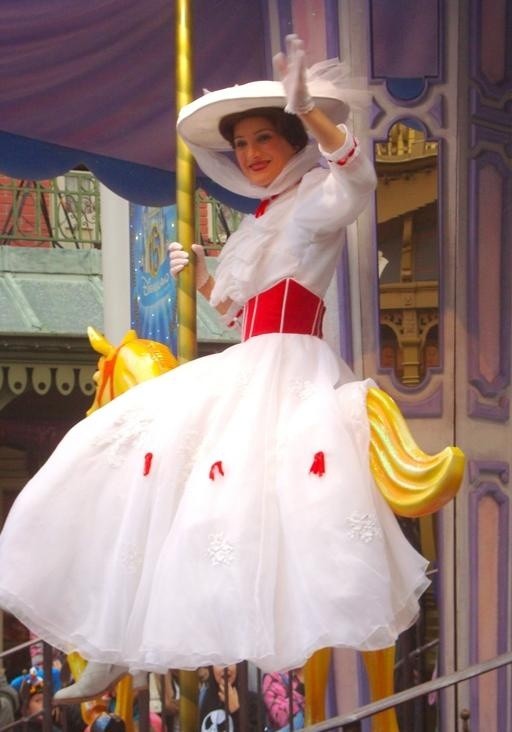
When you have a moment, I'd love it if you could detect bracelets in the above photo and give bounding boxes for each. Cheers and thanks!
[322,133,358,166]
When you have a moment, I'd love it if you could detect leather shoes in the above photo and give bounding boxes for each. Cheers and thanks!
[51,659,130,706]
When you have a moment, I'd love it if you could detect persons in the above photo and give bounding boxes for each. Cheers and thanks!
[1,32,433,708]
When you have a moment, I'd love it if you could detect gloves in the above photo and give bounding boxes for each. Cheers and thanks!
[167,241,211,291]
[271,32,316,116]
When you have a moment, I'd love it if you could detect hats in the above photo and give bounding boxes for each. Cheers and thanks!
[176,79,352,153]
[18,675,44,718]
